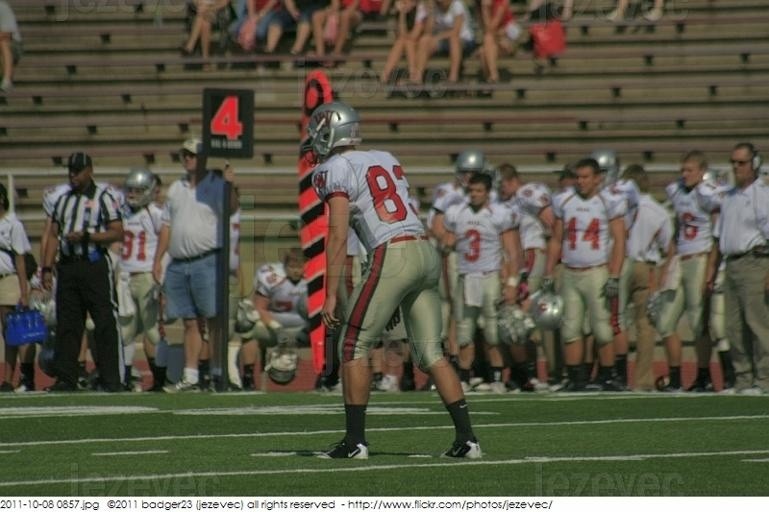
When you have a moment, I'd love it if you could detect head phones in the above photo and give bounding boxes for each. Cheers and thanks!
[752,145,761,170]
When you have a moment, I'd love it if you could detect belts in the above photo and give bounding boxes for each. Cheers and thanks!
[172,247,224,264]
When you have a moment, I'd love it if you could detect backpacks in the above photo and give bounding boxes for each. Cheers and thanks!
[2,303,48,347]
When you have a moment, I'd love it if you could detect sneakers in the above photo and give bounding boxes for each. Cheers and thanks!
[103,382,133,393]
[431,439,481,461]
[161,377,200,394]
[316,439,369,462]
[244,375,258,391]
[13,383,37,393]
[146,384,162,393]
[42,381,78,393]
[77,377,94,391]
[0,382,15,393]
[314,372,769,396]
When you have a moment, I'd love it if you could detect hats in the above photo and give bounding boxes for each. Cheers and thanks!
[179,138,208,154]
[63,152,94,169]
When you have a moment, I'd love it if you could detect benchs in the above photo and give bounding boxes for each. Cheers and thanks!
[1,0,768,390]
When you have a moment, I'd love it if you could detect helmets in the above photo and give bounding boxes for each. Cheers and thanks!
[264,345,301,386]
[300,100,363,167]
[454,150,487,187]
[589,146,619,188]
[234,297,259,344]
[497,302,537,346]
[123,166,157,207]
[529,292,563,331]
